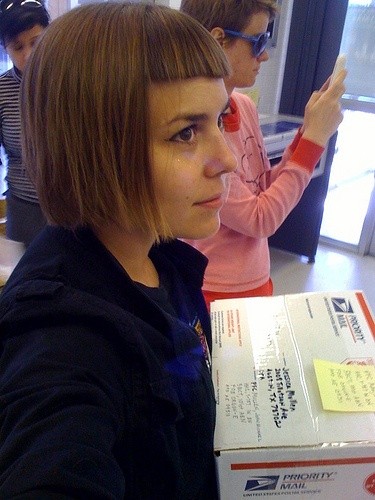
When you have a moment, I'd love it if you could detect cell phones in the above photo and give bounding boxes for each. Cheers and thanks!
[329,54,347,90]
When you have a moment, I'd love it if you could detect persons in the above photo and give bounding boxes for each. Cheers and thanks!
[0,0,54,247]
[177,0,347,313]
[0,0,242,500]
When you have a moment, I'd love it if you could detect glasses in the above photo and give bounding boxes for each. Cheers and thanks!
[223,29,271,57]
[0,0,43,14]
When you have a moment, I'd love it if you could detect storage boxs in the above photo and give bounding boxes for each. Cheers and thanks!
[209,289,375,500]
